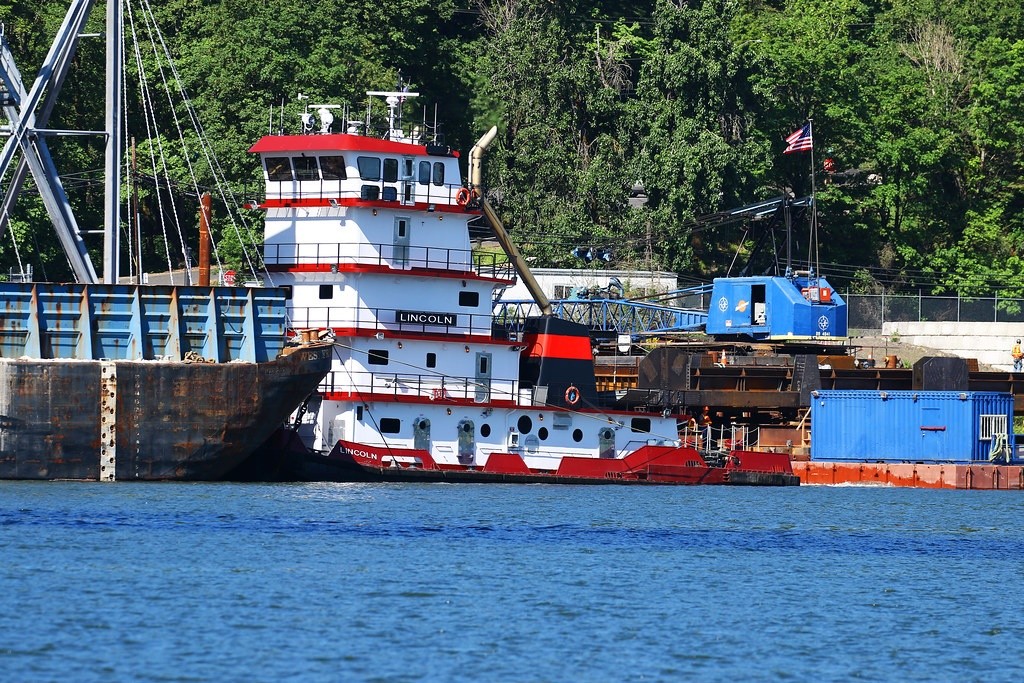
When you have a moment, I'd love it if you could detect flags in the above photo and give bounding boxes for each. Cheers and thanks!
[783,124,812,154]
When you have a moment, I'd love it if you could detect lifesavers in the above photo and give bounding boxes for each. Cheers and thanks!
[565,386,580,405]
[456,188,470,206]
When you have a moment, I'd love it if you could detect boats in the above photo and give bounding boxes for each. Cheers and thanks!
[244,68,803,488]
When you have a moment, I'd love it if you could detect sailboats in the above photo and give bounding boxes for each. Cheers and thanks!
[1,0,339,486]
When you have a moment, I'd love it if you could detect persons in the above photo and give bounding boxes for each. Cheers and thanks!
[1011,339,1024,372]
[698,406,712,449]
